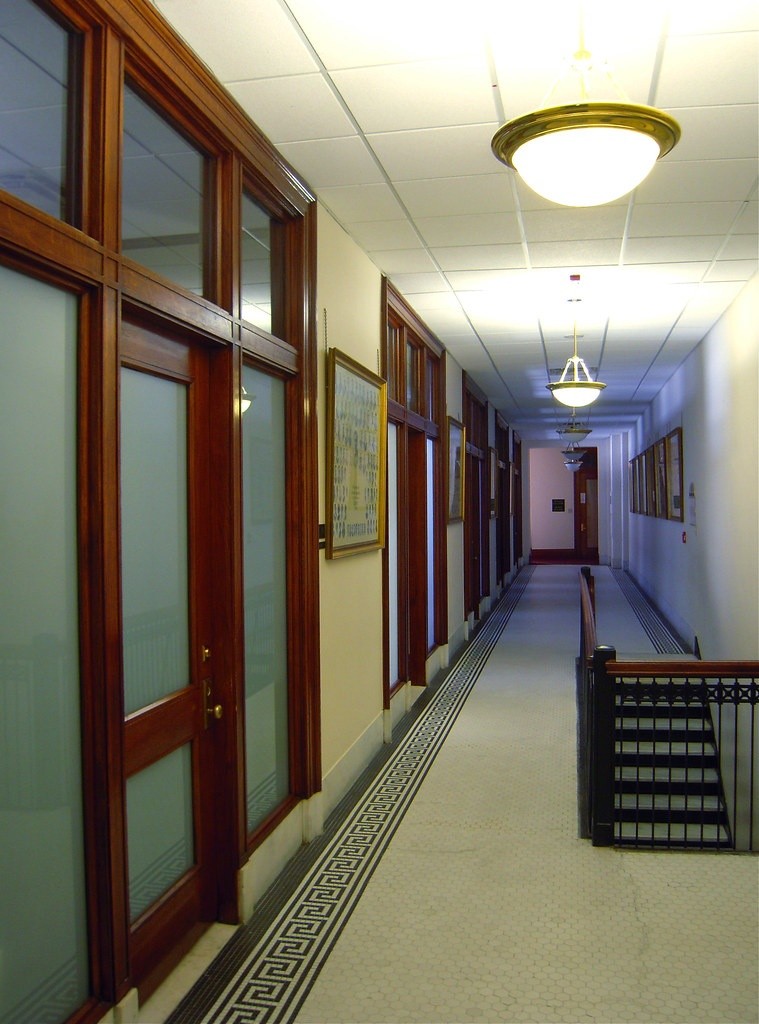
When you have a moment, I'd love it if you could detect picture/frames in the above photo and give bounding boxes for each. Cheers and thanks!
[628,425,685,523]
[325,346,514,560]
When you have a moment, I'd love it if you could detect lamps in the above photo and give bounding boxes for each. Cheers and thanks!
[561,442,586,460]
[491,34,681,207]
[555,407,593,443]
[563,458,583,472]
[544,324,607,408]
